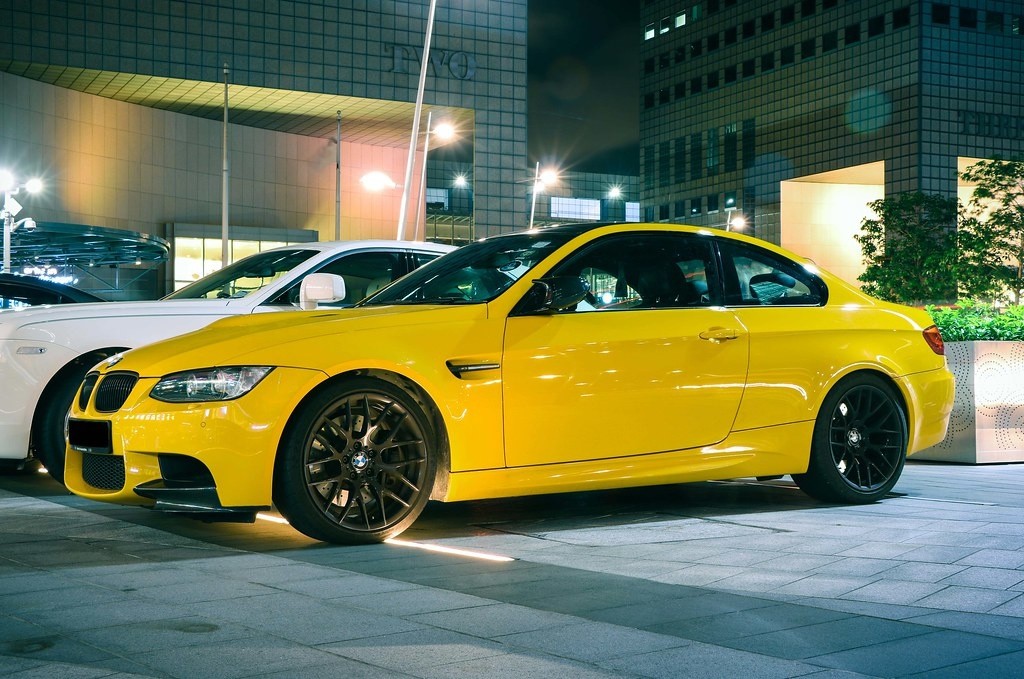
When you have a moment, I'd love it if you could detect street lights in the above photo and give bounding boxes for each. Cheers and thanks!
[413,111,450,242]
[611,187,620,220]
[529,161,555,229]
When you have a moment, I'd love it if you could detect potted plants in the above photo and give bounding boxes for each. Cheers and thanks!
[906,294,1023,465]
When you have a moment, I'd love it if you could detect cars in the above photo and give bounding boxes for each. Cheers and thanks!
[0,239,595,487]
[62,221,957,547]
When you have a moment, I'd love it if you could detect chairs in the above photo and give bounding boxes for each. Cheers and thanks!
[365,276,391,297]
[462,261,796,312]
